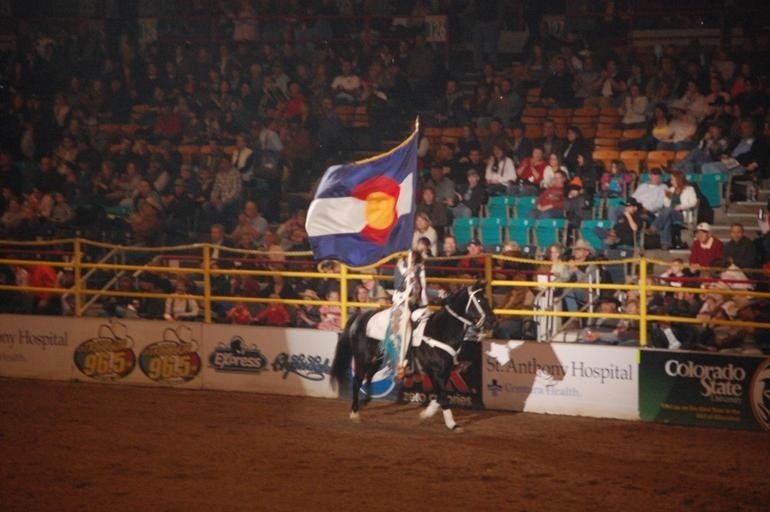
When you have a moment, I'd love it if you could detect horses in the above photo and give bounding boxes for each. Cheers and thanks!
[329,280,499,435]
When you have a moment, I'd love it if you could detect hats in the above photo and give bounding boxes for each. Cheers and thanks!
[696,221,712,232]
[570,238,598,257]
[418,210,434,226]
[298,288,322,302]
[592,293,622,306]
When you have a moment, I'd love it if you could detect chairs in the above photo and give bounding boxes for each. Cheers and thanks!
[421,87,729,252]
